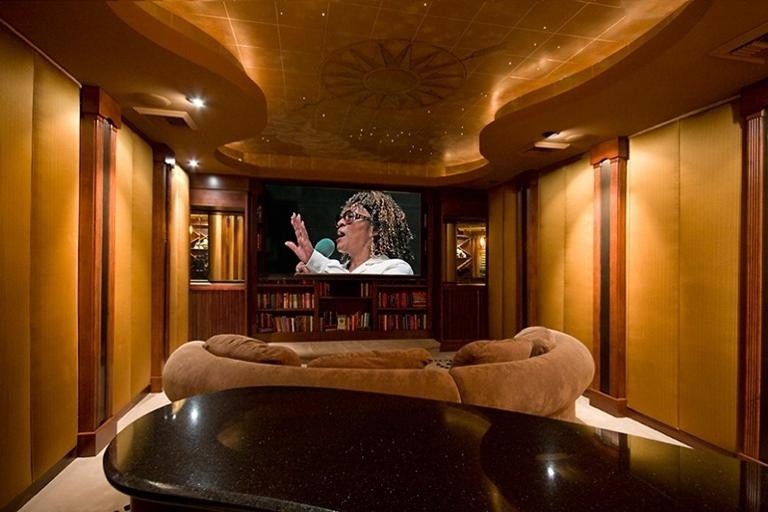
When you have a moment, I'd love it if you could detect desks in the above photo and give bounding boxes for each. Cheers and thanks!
[103,386,767,511]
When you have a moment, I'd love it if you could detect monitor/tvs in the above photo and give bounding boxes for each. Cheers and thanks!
[260,179,429,285]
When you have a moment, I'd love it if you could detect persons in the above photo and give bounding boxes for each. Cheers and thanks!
[284,191,416,276]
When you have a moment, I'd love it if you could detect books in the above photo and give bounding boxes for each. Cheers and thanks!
[256,280,427,333]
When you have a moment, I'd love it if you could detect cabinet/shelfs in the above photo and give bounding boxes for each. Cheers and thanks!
[252,282,433,337]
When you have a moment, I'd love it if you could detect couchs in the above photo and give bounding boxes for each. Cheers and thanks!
[161,324,596,423]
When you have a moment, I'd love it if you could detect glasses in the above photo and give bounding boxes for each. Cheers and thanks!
[335,210,375,225]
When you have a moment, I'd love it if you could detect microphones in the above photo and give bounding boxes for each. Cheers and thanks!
[315,238,336,258]
